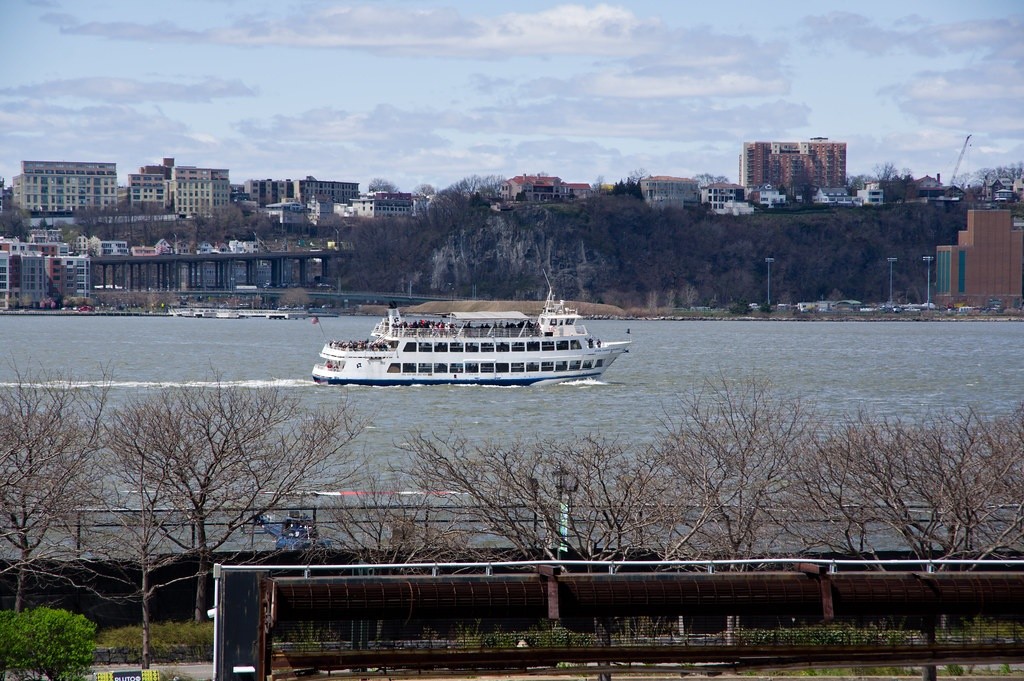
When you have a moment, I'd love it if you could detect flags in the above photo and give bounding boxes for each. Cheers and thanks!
[311,317,319,324]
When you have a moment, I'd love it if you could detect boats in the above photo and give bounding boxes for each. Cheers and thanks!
[311,268,633,387]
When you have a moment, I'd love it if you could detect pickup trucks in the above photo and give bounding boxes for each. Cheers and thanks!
[905,307,920,312]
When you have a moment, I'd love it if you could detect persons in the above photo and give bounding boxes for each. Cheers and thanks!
[327,338,388,372]
[392,317,541,338]
[588,338,593,349]
[597,339,601,352]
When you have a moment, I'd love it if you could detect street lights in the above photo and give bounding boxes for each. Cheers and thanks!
[923,256,934,311]
[887,258,898,303]
[765,258,776,304]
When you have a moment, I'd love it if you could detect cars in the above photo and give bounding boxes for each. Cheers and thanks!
[275,304,304,311]
[315,282,334,290]
[78,305,95,311]
[256,282,273,289]
